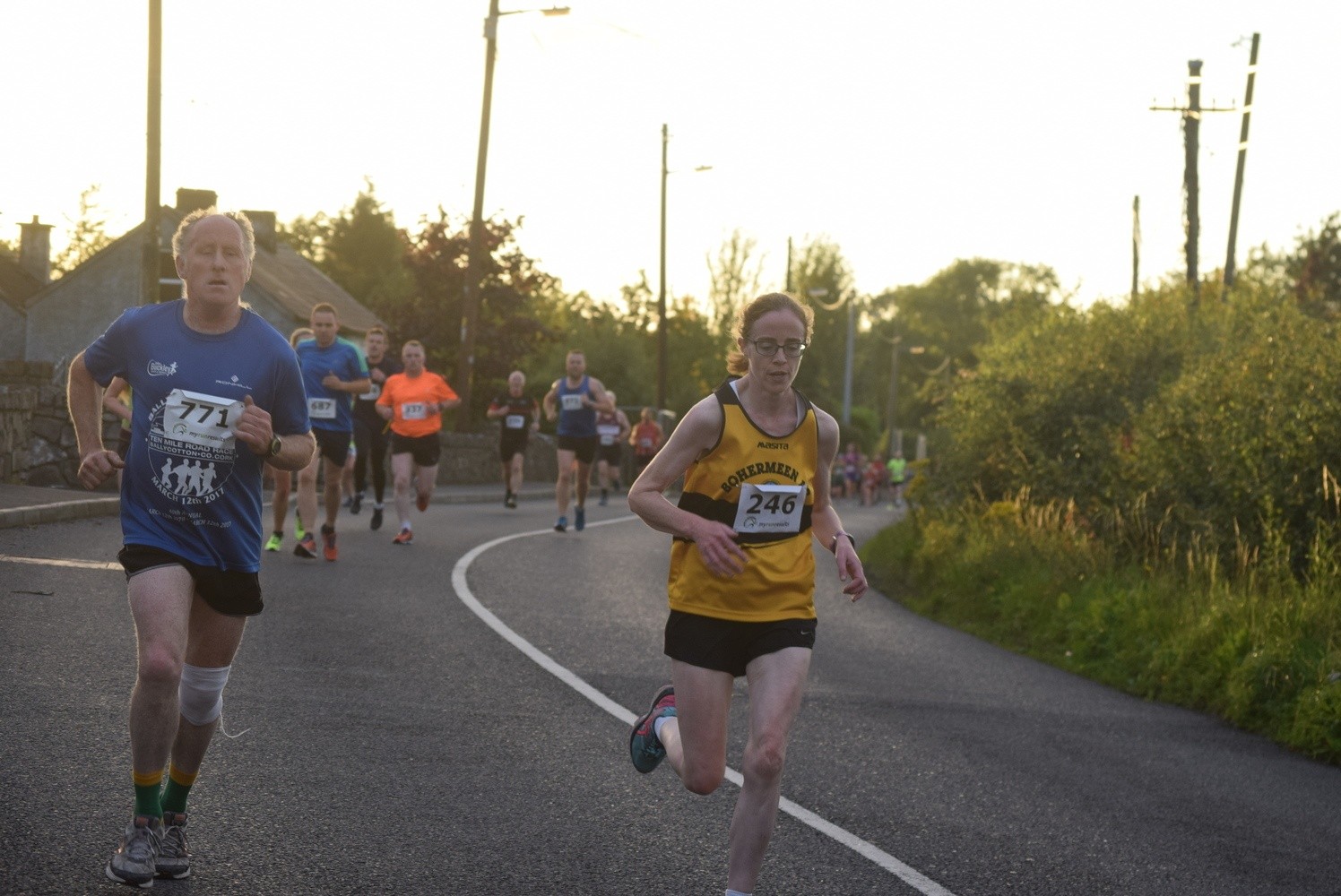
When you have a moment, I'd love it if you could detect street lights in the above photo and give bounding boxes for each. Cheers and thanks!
[653,122,715,410]
[842,297,905,428]
[455,1,572,436]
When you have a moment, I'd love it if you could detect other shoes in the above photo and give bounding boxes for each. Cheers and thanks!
[554,516,568,531]
[599,493,608,505]
[503,488,517,508]
[343,497,352,508]
[371,509,384,530]
[574,504,584,530]
[351,494,364,514]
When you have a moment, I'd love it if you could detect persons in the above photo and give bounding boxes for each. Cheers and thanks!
[594,389,632,509]
[829,440,910,509]
[374,339,461,545]
[261,304,371,562]
[627,407,664,475]
[486,370,541,510]
[67,209,316,888]
[543,349,613,533]
[629,292,870,896]
[351,319,398,531]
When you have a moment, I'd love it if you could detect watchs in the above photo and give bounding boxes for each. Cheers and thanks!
[829,531,856,553]
[265,433,281,459]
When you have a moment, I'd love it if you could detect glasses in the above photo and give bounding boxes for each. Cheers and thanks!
[747,338,807,358]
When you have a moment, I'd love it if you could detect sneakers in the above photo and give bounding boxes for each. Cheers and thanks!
[293,504,305,541]
[414,476,430,511]
[294,532,318,559]
[154,811,193,880]
[264,533,282,552]
[321,524,338,561]
[393,527,413,545]
[106,813,163,888]
[630,684,678,774]
